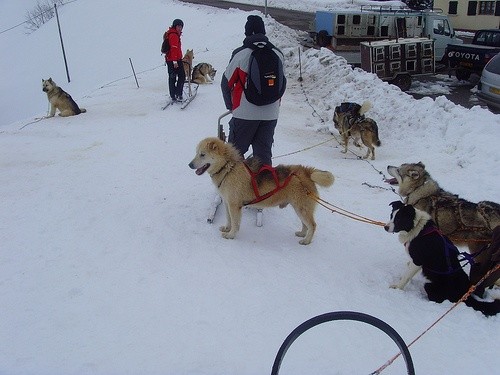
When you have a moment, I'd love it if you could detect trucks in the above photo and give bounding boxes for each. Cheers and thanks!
[315,5,457,70]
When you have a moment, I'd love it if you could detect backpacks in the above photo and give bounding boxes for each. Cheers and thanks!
[239,42,287,107]
[160,32,178,54]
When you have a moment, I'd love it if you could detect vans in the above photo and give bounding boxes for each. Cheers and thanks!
[476,52,500,112]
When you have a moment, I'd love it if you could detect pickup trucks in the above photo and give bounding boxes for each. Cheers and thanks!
[447,27,500,80]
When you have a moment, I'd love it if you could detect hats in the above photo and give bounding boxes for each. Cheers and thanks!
[245,14,266,36]
[173,18,183,27]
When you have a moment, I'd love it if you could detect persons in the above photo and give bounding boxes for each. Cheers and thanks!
[222,15,287,168]
[163,19,186,101]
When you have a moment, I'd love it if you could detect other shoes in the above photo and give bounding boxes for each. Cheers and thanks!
[171,94,187,102]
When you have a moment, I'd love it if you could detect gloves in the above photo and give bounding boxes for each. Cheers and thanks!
[173,60,178,69]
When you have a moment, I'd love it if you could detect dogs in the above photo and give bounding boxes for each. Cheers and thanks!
[188,136,335,246]
[42,77,86,117]
[332,110,381,160]
[180,49,217,84]
[384,161,500,318]
